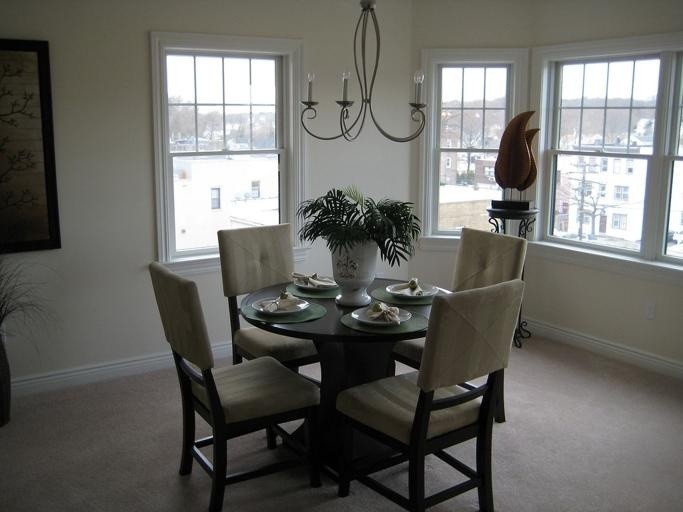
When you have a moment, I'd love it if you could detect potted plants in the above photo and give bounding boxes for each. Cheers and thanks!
[294,184,422,306]
[0,252,66,428]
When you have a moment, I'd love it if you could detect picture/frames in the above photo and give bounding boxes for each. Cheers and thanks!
[1,38,61,254]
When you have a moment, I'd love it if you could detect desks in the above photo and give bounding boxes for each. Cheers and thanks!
[486,210,542,349]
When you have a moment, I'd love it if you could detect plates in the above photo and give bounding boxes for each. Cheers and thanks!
[293,276,338,292]
[350,305,411,327]
[385,283,439,300]
[251,296,309,316]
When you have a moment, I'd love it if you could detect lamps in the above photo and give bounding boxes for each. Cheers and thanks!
[299,0,429,143]
